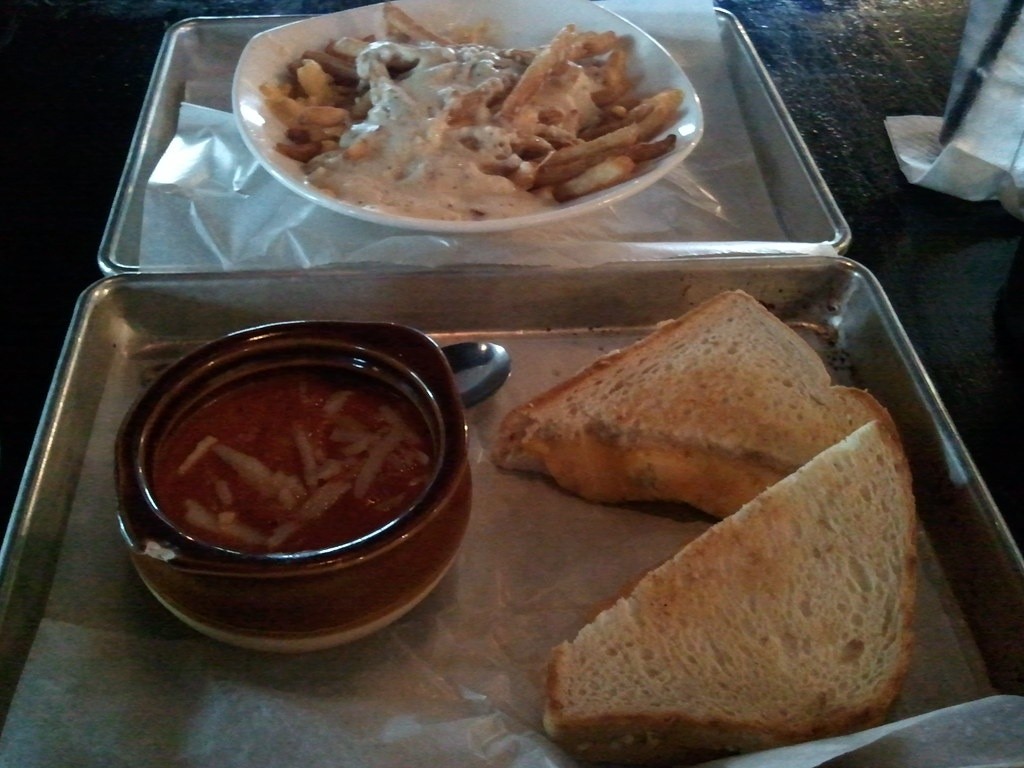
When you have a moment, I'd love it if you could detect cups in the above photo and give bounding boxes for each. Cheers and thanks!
[938,1,1024,174]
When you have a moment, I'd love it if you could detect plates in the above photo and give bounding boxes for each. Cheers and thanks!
[129,459,475,650]
[0,261,1024,768]
[232,2,705,235]
[95,9,853,259]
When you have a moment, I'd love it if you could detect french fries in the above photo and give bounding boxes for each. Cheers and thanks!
[259,0,685,203]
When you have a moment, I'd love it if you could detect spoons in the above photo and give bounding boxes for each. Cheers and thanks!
[136,339,513,407]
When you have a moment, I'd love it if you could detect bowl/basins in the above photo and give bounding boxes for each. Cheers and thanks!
[112,321,465,586]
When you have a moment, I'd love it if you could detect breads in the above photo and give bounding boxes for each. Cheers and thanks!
[498,287,916,761]
